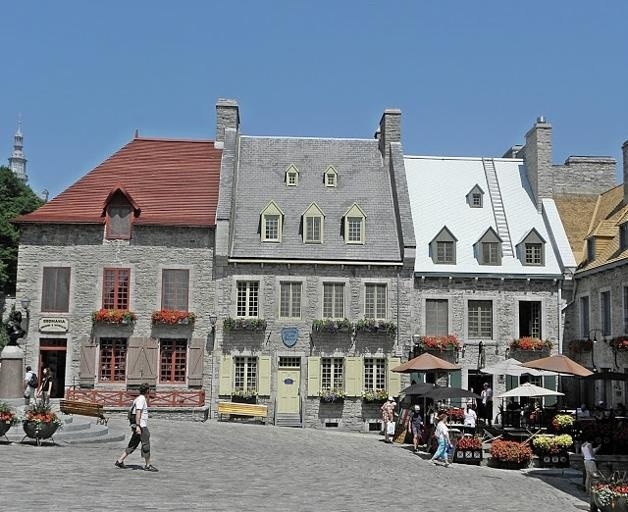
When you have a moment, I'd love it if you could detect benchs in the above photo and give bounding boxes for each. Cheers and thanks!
[218,402,269,424]
[58,399,111,427]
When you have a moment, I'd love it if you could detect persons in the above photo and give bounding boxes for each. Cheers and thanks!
[463,382,493,434]
[37,367,53,406]
[581,436,602,492]
[380,397,399,443]
[115,382,158,472]
[408,404,453,467]
[571,401,624,419]
[24,366,38,405]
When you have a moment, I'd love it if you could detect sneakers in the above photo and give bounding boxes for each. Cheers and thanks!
[144,465,159,472]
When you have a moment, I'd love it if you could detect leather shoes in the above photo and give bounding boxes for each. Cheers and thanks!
[115,460,128,469]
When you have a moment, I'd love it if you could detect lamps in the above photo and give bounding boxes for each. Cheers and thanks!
[209,311,216,332]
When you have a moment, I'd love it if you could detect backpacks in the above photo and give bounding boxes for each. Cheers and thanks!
[27,372,38,388]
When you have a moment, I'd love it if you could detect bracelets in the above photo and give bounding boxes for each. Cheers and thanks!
[136,424,141,427]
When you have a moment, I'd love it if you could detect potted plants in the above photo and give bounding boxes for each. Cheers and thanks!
[217,315,267,334]
[309,316,398,342]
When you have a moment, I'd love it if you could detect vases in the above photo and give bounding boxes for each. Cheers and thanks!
[319,397,344,405]
[231,396,256,405]
[0,417,59,447]
[363,397,387,405]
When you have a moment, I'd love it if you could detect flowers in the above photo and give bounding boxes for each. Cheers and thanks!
[362,389,389,400]
[151,307,196,329]
[610,334,627,353]
[446,409,578,469]
[316,387,347,404]
[89,308,136,343]
[511,337,553,352]
[228,386,257,398]
[421,333,461,355]
[0,391,65,435]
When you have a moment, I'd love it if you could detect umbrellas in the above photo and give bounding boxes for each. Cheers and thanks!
[390,352,595,424]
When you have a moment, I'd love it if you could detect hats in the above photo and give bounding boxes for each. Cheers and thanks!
[412,404,421,411]
[389,397,395,401]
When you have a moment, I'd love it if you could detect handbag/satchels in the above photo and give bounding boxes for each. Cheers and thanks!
[127,404,136,424]
[386,421,395,435]
